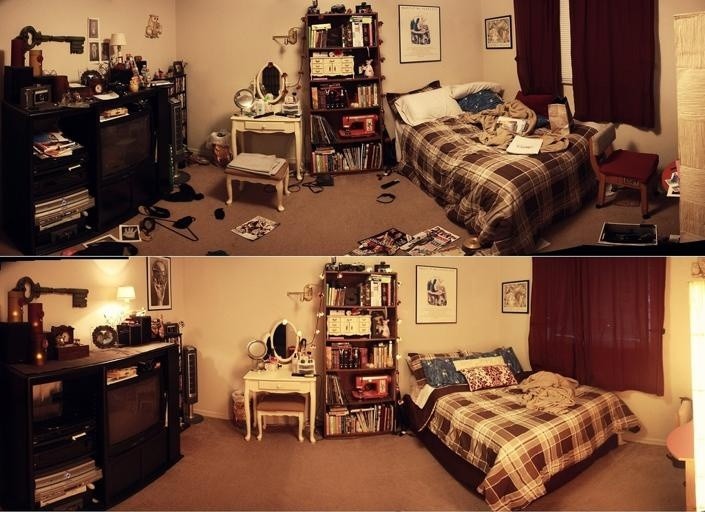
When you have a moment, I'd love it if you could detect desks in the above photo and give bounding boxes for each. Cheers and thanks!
[666,417,695,512]
[242,367,316,443]
[230,110,302,181]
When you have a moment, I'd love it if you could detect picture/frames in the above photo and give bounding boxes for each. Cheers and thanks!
[88,41,100,64]
[502,280,529,314]
[146,257,172,310]
[398,4,441,64]
[100,41,110,62]
[415,265,458,325]
[87,17,99,41]
[485,15,512,49]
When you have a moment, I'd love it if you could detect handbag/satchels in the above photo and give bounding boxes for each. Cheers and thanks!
[302,173,334,194]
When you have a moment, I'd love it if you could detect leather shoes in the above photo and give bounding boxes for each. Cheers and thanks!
[147,203,169,217]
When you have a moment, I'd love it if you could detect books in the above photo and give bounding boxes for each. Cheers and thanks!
[230,216,281,241]
[312,84,350,109]
[507,135,543,155]
[665,172,680,196]
[312,144,384,172]
[310,115,337,144]
[326,284,346,306]
[33,129,82,159]
[326,405,394,436]
[369,342,394,369]
[326,342,368,369]
[345,226,466,255]
[308,23,331,48]
[325,375,344,405]
[354,85,378,108]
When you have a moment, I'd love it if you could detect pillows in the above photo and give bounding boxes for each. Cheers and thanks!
[458,90,505,113]
[420,358,466,387]
[459,365,518,392]
[449,81,505,99]
[466,346,505,359]
[482,347,526,379]
[396,87,462,126]
[386,80,442,125]
[406,351,464,389]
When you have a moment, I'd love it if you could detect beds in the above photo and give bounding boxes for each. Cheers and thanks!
[414,381,641,512]
[382,94,606,256]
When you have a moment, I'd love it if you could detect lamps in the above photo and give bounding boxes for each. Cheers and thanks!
[272,28,297,54]
[287,285,313,303]
[109,33,128,64]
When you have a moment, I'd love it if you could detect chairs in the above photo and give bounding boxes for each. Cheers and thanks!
[589,123,659,220]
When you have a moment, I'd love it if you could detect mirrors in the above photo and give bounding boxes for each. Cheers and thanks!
[257,63,285,104]
[271,320,300,362]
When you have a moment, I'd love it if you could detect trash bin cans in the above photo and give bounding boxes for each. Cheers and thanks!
[210,131,231,166]
[232,390,253,431]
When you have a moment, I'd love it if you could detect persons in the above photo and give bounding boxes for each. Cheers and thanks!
[151,261,169,306]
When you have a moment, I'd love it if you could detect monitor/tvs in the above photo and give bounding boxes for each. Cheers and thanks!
[99,109,156,184]
[106,368,166,456]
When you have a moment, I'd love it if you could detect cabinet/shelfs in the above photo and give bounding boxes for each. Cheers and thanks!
[305,12,385,177]
[322,270,399,439]
[0,86,173,257]
[0,340,184,512]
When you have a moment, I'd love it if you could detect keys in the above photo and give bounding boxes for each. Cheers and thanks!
[20,26,85,54]
[17,276,89,308]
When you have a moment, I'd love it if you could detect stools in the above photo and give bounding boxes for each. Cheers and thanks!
[256,394,306,442]
[225,164,291,212]
